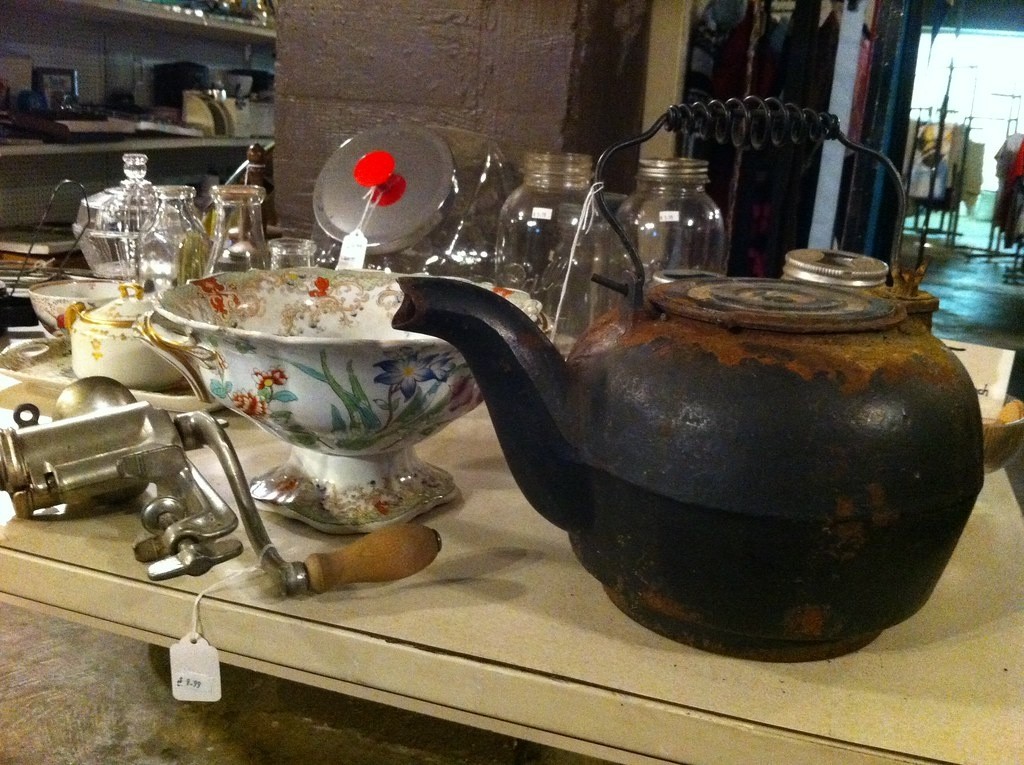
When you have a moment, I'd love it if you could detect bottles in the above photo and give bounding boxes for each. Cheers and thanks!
[70,144,887,360]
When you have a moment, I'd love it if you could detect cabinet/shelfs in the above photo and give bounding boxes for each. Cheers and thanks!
[2,1,274,277]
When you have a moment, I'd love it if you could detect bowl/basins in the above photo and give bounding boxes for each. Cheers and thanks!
[134,266,543,536]
[27,279,136,354]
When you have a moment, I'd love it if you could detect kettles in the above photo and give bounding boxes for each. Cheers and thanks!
[391,95,986,662]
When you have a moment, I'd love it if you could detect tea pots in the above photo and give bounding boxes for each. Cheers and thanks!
[67,282,188,394]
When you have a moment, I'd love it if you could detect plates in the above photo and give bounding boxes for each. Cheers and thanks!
[0,336,227,416]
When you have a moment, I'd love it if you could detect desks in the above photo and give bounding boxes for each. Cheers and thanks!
[1,277,1024,764]
